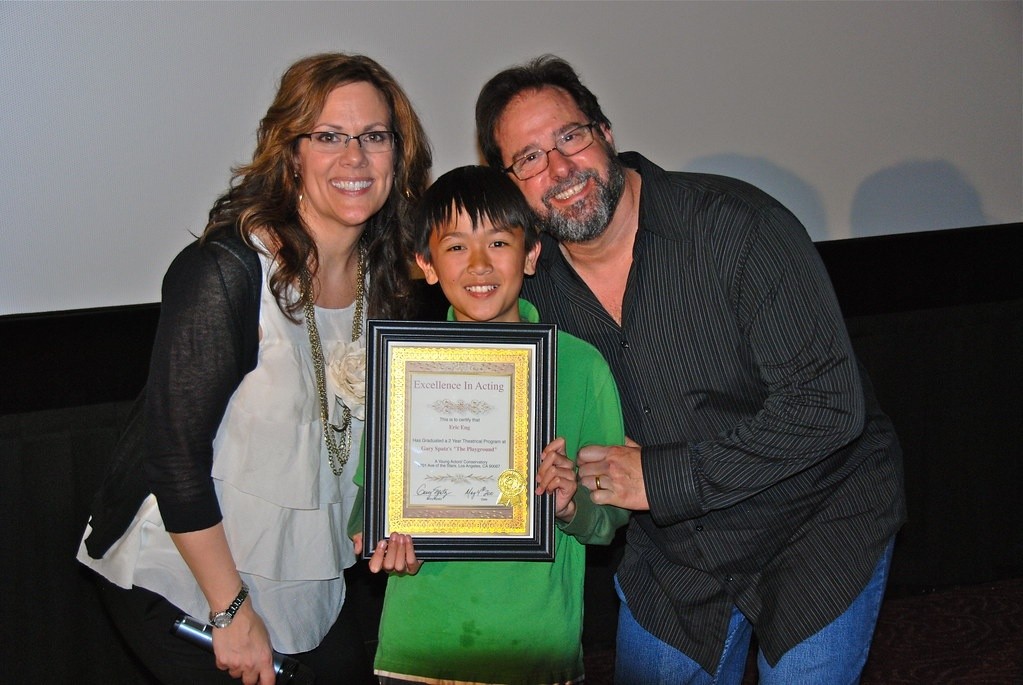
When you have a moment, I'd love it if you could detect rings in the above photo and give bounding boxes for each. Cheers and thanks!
[595,474,601,489]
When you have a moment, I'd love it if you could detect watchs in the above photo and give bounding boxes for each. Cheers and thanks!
[209,578,250,628]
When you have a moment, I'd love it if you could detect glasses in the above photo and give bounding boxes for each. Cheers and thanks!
[297,128,397,153]
[502,120,599,181]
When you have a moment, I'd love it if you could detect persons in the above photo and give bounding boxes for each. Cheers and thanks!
[72,53,433,685]
[475,52,907,683]
[347,163,635,684]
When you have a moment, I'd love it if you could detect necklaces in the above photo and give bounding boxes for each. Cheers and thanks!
[298,236,369,476]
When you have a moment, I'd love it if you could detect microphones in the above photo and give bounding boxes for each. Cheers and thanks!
[168,614,316,685]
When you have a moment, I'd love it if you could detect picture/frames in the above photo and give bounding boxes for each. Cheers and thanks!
[363,319,557,563]
